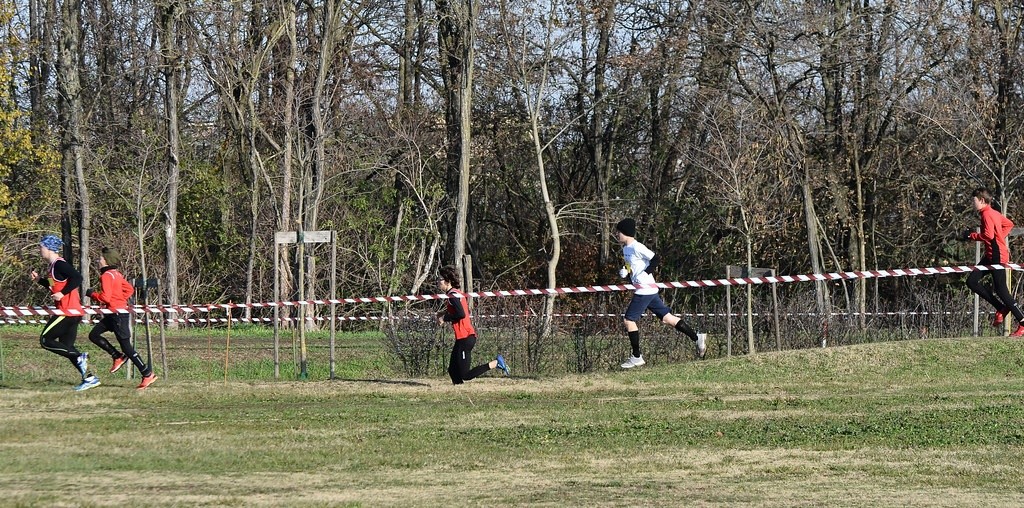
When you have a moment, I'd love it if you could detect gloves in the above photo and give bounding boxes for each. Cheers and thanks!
[634,271,649,285]
[955,230,973,242]
[618,266,628,278]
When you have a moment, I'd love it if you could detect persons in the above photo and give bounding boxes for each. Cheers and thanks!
[434,269,511,385]
[30,235,101,392]
[84,249,159,390]
[965,187,1024,337]
[613,218,707,368]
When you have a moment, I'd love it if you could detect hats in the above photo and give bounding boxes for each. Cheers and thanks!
[101,246,120,266]
[617,217,636,237]
[40,235,63,252]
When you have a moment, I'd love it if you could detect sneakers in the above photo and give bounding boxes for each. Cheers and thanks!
[75,352,90,375]
[495,354,510,376]
[74,374,100,392]
[621,354,646,369]
[695,332,706,357]
[992,304,1010,326]
[136,373,158,390]
[109,352,130,373]
[1010,324,1024,338]
